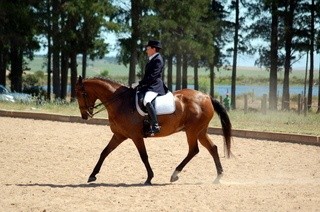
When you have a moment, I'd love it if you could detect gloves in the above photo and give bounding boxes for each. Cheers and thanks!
[127,89,136,96]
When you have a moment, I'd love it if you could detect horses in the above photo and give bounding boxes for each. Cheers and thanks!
[75,75,232,185]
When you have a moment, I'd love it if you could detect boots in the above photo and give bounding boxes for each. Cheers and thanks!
[145,102,160,134]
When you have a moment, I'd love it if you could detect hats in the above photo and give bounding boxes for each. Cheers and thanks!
[146,40,162,49]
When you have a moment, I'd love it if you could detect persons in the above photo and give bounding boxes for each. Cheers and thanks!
[133,40,166,135]
